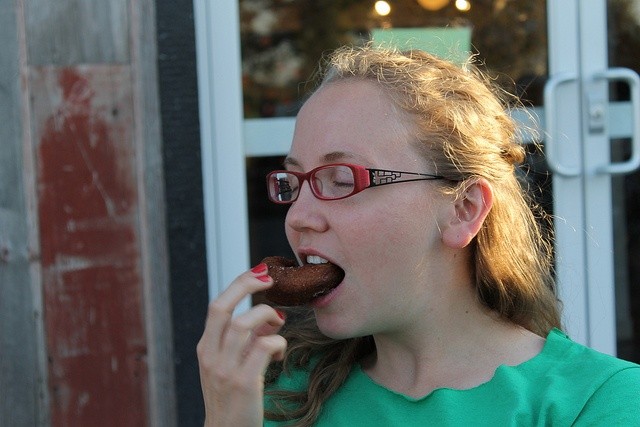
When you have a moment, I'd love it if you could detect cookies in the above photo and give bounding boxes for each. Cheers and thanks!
[256,256,343,307]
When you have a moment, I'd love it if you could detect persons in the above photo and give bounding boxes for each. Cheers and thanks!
[196,45,639,427]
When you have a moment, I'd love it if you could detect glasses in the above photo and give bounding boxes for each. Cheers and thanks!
[267,164,446,203]
